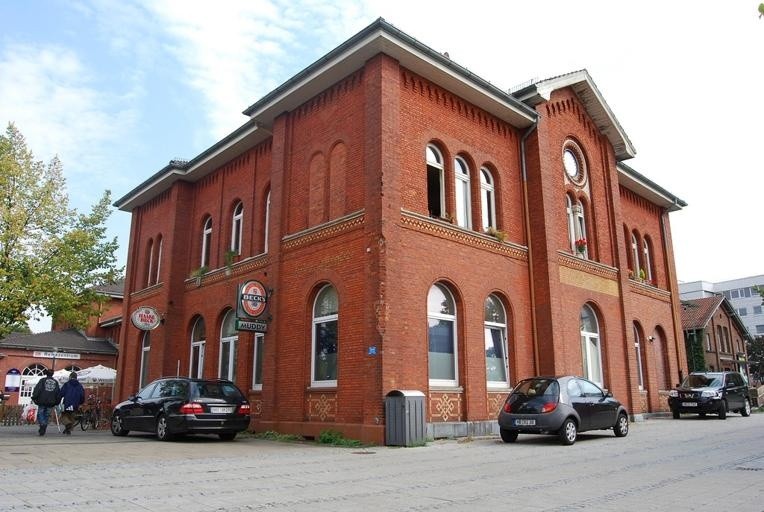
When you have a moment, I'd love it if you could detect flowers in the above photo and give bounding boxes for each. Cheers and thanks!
[575,237,586,251]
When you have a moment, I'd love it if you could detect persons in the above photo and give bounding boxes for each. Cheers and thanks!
[59,372,85,433]
[32,369,60,435]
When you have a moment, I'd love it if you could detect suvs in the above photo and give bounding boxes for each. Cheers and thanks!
[667,370,755,422]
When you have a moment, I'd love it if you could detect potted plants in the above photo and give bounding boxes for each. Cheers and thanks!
[223,248,240,269]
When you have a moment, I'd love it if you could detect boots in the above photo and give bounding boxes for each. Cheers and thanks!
[37,422,74,436]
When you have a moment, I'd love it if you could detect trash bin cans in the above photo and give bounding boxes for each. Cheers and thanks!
[384,389,426,446]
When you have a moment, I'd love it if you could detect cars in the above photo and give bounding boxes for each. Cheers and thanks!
[107,375,254,442]
[496,372,631,448]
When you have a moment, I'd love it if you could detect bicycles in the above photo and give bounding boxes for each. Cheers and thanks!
[72,399,102,430]
[80,392,103,432]
